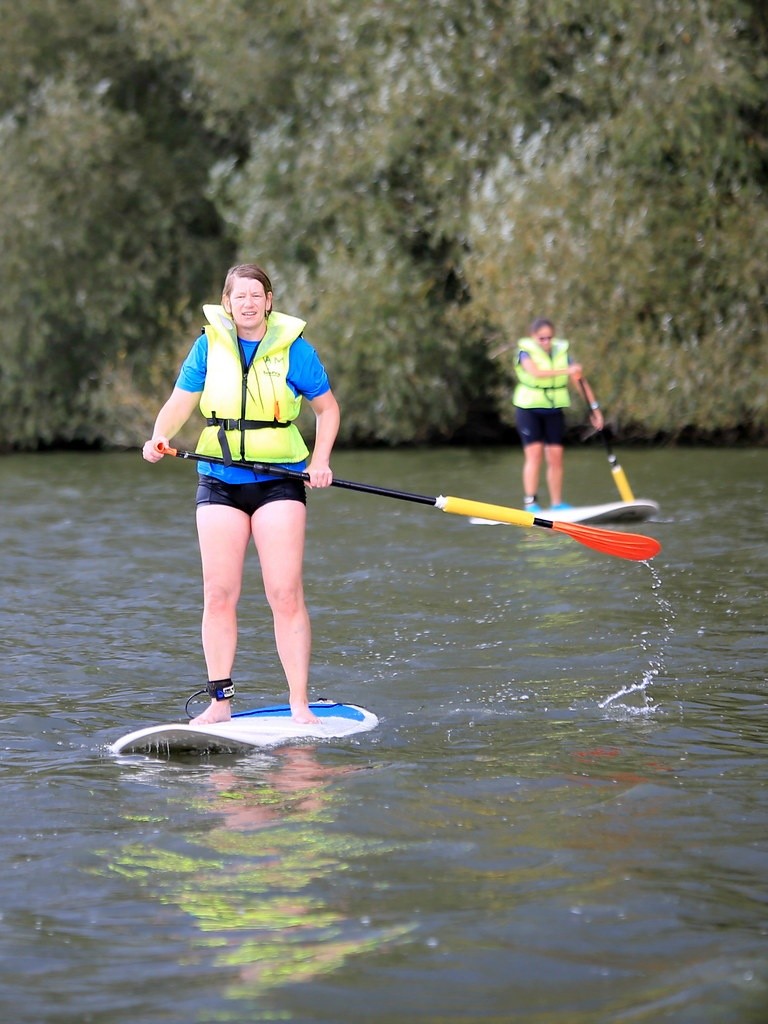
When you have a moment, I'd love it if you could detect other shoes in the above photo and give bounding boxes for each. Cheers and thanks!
[523,503,544,513]
[550,502,575,510]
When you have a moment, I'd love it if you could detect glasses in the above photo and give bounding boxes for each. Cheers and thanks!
[535,333,552,341]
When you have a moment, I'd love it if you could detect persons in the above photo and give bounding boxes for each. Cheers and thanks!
[512,315,606,515]
[141,262,343,730]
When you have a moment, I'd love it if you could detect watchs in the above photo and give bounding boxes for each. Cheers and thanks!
[589,401,600,411]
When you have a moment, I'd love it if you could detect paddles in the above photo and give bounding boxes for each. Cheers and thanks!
[152,441,665,567]
[564,346,639,503]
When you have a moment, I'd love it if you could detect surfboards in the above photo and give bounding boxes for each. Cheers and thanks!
[105,696,381,758]
[459,495,659,527]
